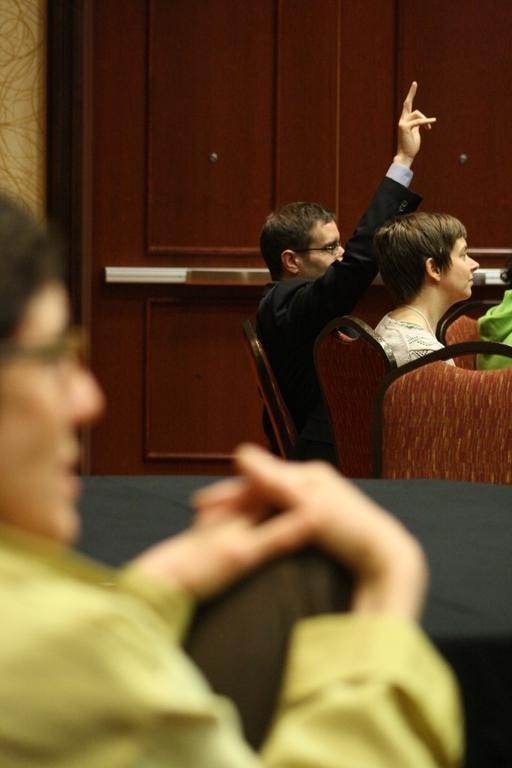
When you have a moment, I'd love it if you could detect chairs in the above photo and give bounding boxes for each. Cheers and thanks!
[434,298,507,368]
[372,339,512,484]
[313,313,397,473]
[241,314,306,471]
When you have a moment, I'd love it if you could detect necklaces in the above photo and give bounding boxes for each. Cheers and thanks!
[401,301,435,337]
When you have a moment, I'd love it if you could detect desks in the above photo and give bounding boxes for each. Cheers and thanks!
[72,473,512,766]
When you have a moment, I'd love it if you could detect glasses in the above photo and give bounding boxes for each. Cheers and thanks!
[0,325,92,392]
[292,241,341,259]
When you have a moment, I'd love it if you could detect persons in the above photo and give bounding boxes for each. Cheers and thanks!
[475,267,511,371]
[372,211,480,370]
[250,78,436,465]
[0,187,466,767]
